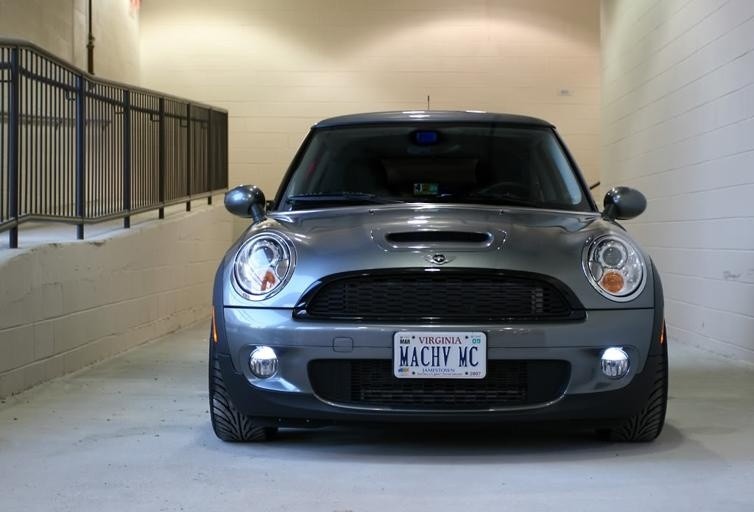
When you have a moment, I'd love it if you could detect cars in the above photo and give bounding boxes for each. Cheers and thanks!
[208,100,667,445]
